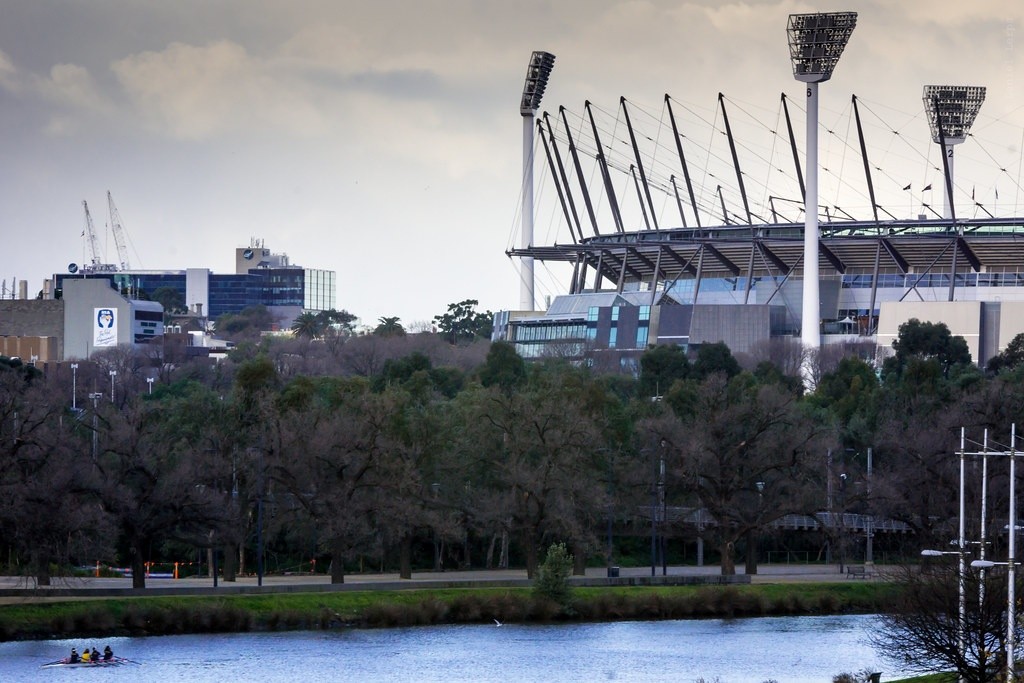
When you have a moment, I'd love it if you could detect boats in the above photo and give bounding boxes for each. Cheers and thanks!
[38,656,128,670]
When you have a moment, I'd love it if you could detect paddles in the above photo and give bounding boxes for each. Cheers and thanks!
[78,654,142,668]
[43,656,83,666]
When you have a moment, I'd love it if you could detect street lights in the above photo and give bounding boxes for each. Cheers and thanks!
[824,446,855,512]
[919,550,972,683]
[517,49,560,310]
[70,363,79,409]
[922,83,989,221]
[784,9,857,395]
[147,377,153,394]
[246,446,265,587]
[948,540,993,683]
[89,393,104,471]
[754,480,765,513]
[640,447,656,576]
[109,370,117,404]
[968,559,1022,683]
[597,447,613,568]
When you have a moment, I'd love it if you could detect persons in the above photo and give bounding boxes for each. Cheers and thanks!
[70,647,81,663]
[81,648,91,662]
[91,646,101,661]
[103,646,113,660]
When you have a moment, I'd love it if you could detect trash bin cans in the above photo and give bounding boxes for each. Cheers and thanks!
[607,567,620,577]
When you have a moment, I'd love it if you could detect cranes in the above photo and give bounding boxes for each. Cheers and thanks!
[80,199,102,266]
[105,188,132,271]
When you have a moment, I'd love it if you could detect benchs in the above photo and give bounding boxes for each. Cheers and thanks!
[847,565,873,579]
